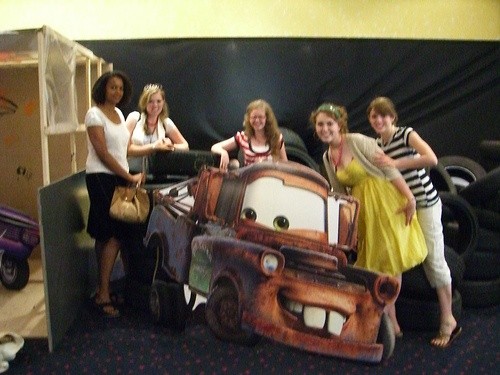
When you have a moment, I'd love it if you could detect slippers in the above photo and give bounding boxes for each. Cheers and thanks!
[92,294,120,318]
[431,326,463,350]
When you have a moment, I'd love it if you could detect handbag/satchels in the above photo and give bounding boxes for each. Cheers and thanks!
[109,176,150,223]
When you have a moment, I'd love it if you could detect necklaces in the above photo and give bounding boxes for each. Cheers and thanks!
[380,125,394,151]
[326,135,344,173]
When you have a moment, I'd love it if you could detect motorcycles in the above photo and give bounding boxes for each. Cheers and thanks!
[0,206,41,291]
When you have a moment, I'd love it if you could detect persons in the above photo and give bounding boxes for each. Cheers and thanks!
[367,96,458,347]
[209,99,289,170]
[85,69,143,318]
[310,101,428,338]
[126,81,189,314]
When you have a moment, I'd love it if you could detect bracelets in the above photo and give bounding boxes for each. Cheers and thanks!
[407,197,416,202]
[152,141,161,152]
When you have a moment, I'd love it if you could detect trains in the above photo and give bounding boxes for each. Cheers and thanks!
[122,128,401,362]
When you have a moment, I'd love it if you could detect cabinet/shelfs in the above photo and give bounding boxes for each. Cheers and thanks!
[0,24,113,225]
